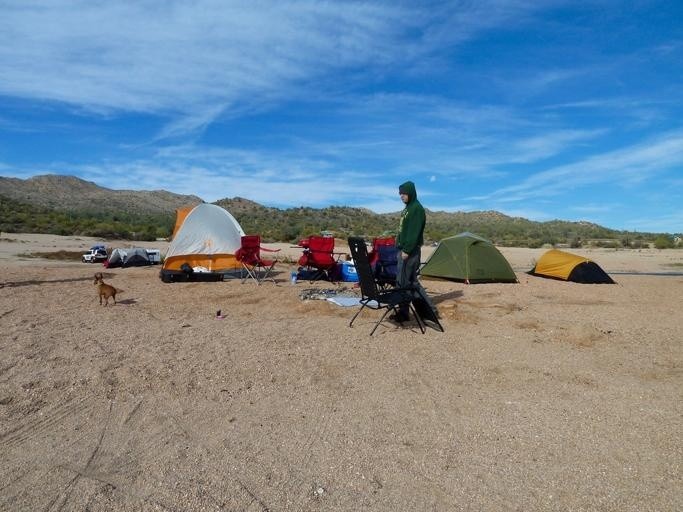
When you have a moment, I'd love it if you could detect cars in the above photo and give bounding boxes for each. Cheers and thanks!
[80,248,108,263]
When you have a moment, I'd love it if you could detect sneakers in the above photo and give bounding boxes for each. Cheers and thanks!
[390,313,409,322]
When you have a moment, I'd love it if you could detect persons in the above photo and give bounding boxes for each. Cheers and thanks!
[390,181,436,322]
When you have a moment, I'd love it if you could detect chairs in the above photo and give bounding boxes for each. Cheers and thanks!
[299,237,346,285]
[348,235,444,335]
[236,236,281,288]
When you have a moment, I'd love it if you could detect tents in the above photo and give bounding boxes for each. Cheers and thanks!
[160,201,247,279]
[527,249,616,283]
[418,232,519,285]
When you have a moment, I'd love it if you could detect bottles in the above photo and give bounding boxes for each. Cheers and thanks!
[290,271,296,285]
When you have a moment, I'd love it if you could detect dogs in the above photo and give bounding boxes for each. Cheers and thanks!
[93,273,124,307]
